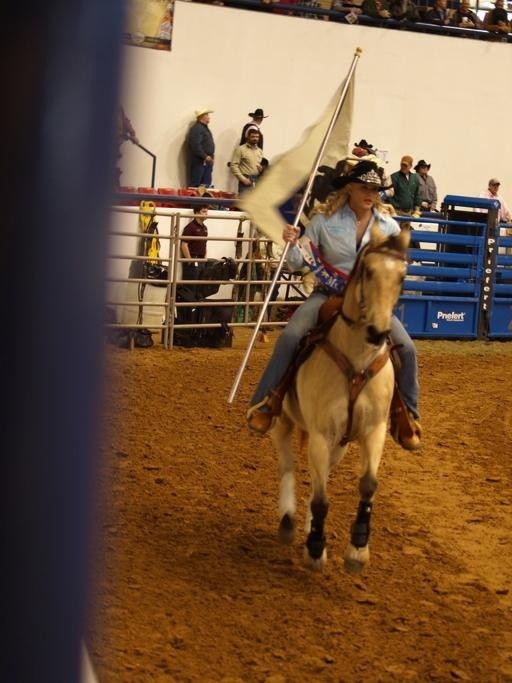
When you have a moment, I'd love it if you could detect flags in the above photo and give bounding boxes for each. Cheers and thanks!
[233,61,357,248]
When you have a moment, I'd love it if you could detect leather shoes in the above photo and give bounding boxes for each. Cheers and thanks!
[249,412,271,432]
[391,417,420,450]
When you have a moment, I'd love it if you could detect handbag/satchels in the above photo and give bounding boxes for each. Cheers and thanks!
[129,262,166,286]
[107,328,153,347]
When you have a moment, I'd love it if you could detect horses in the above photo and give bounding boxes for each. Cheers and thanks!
[268,216,411,575]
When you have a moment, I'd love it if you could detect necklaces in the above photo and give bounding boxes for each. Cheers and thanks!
[356,218,361,226]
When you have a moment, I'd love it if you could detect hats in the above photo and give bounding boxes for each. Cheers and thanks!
[401,156,413,165]
[195,108,212,117]
[331,161,394,191]
[355,140,372,147]
[249,110,269,119]
[414,160,431,170]
[489,179,500,185]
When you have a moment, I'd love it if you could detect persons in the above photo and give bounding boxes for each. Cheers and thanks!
[382,154,421,262]
[240,108,269,151]
[211,0,511,41]
[245,160,423,451]
[187,104,216,187]
[230,128,268,208]
[473,176,511,223]
[413,158,438,212]
[173,202,209,323]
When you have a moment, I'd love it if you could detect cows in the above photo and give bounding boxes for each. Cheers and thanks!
[200,256,239,298]
